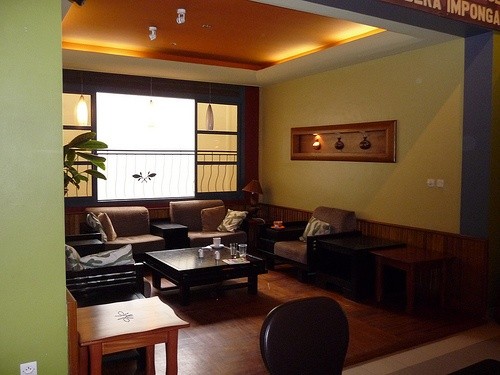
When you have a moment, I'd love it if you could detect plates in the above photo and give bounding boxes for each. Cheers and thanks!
[270,226,285,229]
[210,244,224,248]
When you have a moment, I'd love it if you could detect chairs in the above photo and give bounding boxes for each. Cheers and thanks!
[65,242,146,308]
[169,200,246,250]
[274,206,356,281]
[85,206,165,252]
[260,295,349,375]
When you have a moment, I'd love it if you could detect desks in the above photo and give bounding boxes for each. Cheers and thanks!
[149,221,189,249]
[310,236,406,303]
[370,247,452,312]
[261,220,308,269]
[65,233,104,244]
[143,245,259,302]
[246,216,270,245]
[75,295,189,375]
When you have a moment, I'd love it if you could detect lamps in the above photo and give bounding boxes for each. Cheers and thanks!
[76,71,89,125]
[242,181,264,217]
[205,82,214,131]
[148,26,157,40]
[176,9,186,24]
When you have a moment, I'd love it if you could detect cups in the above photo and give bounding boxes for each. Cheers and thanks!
[273,221,282,228]
[213,237,221,246]
[238,244,247,257]
[230,243,237,255]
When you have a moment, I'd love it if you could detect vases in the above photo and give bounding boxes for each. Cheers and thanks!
[335,138,344,150]
[359,137,371,149]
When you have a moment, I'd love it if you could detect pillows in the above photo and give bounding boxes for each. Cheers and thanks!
[79,244,136,284]
[217,208,248,233]
[300,215,331,242]
[200,206,227,234]
